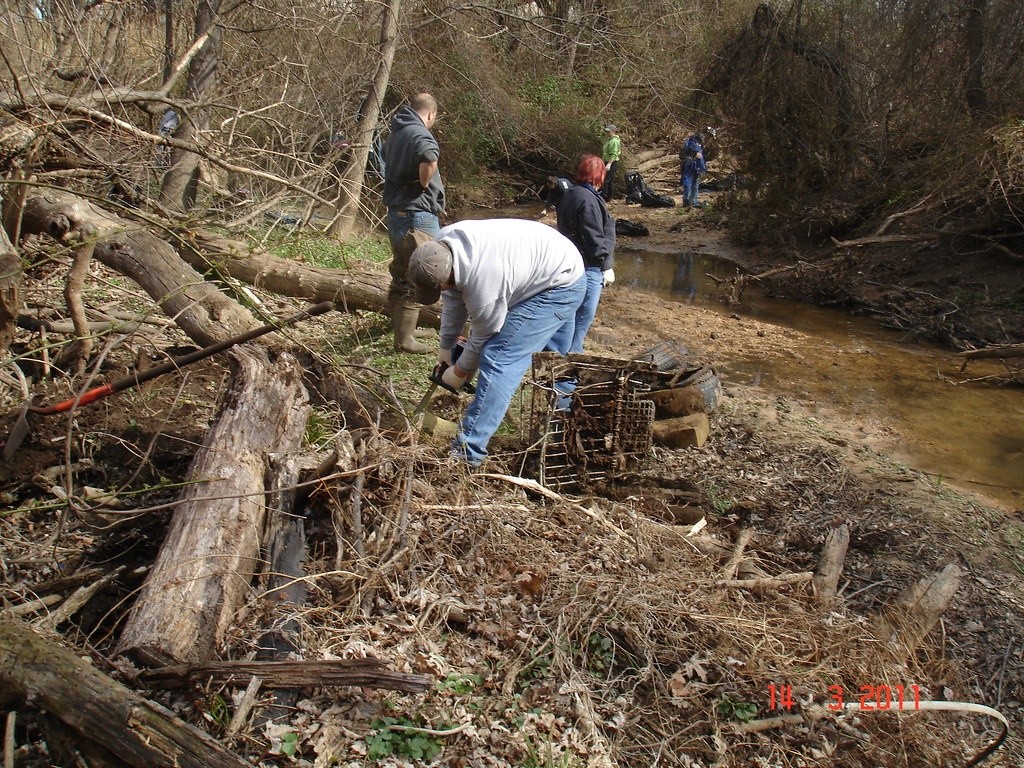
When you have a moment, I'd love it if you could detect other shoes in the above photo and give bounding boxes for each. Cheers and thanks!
[603,198,612,202]
[682,202,692,207]
[693,201,705,208]
[415,449,479,477]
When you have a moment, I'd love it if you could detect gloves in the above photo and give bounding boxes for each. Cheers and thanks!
[541,209,547,215]
[439,348,453,366]
[441,365,469,390]
[605,163,611,171]
[602,268,615,286]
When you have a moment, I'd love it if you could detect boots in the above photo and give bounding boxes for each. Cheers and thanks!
[388,301,435,353]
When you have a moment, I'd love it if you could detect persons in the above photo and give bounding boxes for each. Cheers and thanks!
[540,174,575,216]
[679,135,708,208]
[557,156,616,354]
[379,93,446,355]
[405,218,587,468]
[601,124,622,203]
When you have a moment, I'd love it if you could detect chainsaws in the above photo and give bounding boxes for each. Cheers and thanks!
[413,339,476,416]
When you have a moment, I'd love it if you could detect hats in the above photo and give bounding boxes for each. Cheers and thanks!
[705,126,716,140]
[605,125,617,132]
[409,241,452,305]
